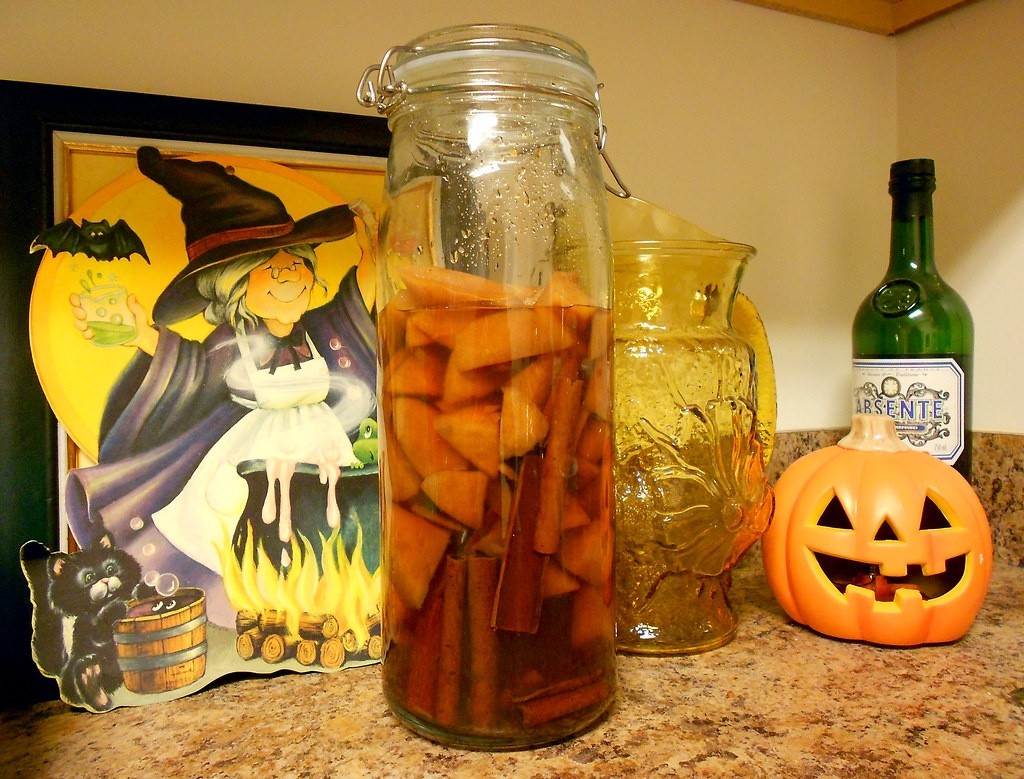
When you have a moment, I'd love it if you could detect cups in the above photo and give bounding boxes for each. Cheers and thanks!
[355,22,632,752]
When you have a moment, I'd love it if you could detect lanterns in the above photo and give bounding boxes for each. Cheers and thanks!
[761,414,992,646]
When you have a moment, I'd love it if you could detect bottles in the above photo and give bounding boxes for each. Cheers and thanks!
[849,159,976,598]
[605,237,779,657]
